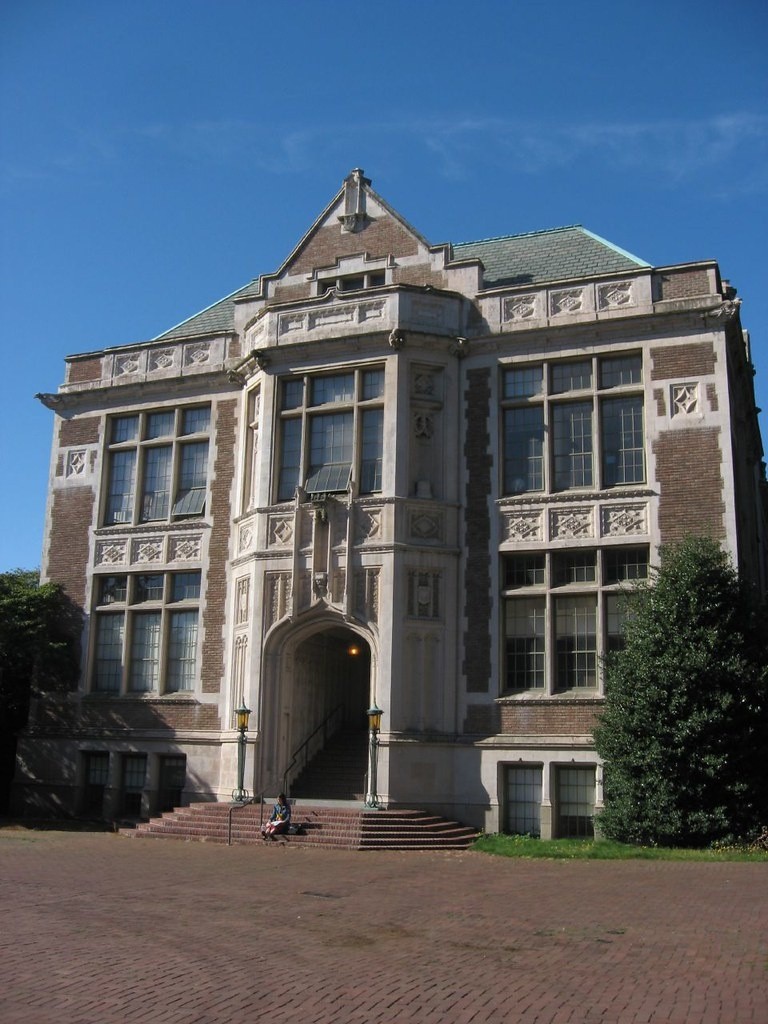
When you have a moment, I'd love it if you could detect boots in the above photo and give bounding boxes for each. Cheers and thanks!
[262,828,275,838]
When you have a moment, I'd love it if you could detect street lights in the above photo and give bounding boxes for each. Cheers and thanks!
[229,696,252,805]
[359,695,387,813]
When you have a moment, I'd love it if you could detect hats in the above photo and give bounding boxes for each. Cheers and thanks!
[277,792,287,798]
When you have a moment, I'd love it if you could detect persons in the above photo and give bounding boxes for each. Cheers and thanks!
[261,792,291,839]
